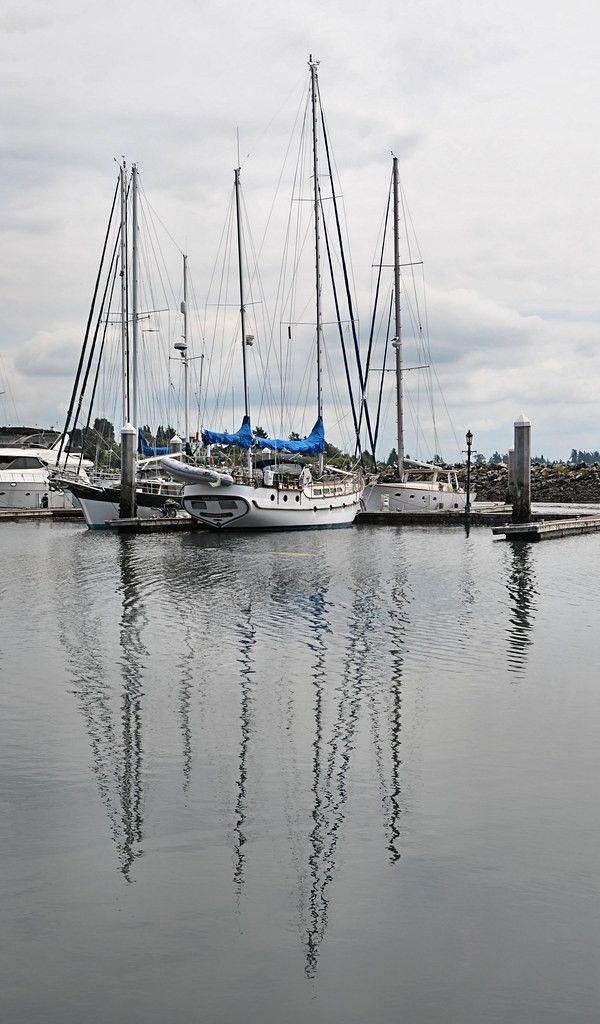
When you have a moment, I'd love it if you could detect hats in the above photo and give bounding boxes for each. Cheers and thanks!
[307,464,312,467]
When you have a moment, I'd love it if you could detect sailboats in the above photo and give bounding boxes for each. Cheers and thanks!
[1,45,480,534]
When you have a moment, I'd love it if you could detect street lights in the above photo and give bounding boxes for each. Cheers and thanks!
[463,427,476,514]
